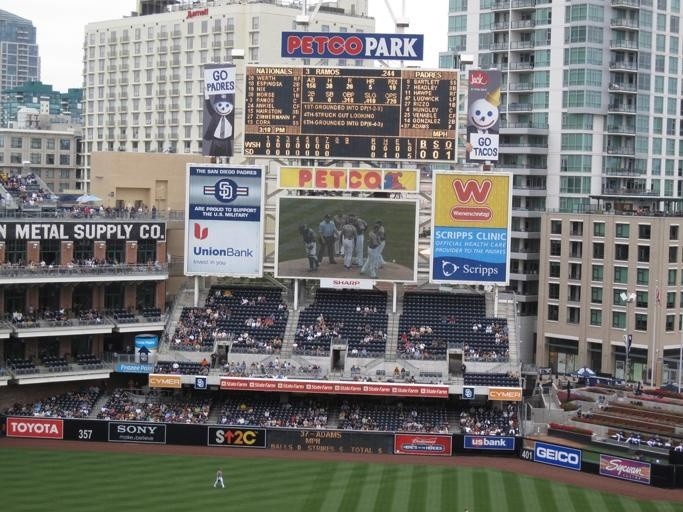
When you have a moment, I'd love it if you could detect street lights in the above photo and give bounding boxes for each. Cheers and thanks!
[620,293,637,383]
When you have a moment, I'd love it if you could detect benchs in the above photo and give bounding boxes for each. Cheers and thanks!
[0,283,520,439]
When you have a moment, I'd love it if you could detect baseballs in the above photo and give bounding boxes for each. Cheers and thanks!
[393,260,395,262]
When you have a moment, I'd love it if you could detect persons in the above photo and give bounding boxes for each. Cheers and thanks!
[214,467,226,488]
[299,210,386,279]
[7,289,521,438]
[576,394,682,451]
[0,172,162,278]
